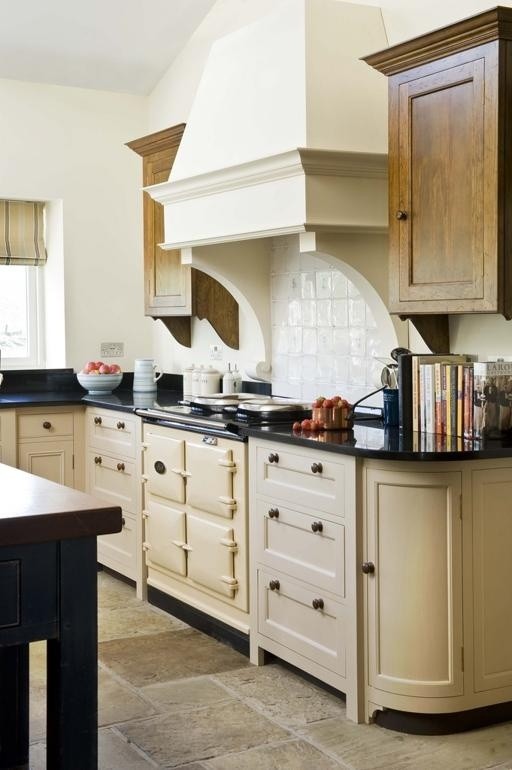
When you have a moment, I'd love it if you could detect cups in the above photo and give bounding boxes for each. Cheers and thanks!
[133,393,156,407]
[383,424,399,453]
[382,388,399,426]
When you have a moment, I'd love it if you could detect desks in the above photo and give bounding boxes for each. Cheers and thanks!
[1,454,121,770]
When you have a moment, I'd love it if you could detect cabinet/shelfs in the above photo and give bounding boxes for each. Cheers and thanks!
[145,144,239,318]
[0,407,16,467]
[249,434,363,694]
[16,405,82,495]
[82,406,143,589]
[389,40,512,315]
[361,455,512,714]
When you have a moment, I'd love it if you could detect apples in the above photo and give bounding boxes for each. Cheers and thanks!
[85,361,120,374]
[312,396,348,408]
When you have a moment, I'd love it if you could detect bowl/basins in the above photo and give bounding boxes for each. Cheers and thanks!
[75,372,123,396]
[83,396,121,405]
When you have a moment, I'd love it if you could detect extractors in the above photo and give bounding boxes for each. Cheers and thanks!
[139,1,390,255]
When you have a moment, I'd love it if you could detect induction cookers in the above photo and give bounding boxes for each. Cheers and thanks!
[134,405,380,441]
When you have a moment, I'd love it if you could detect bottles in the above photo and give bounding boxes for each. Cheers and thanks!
[222,361,243,393]
[130,357,163,393]
[183,364,221,396]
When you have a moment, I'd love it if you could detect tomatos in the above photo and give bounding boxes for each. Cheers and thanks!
[292,418,319,430]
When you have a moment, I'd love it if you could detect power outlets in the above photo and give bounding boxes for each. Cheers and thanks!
[209,344,224,360]
[101,342,124,357]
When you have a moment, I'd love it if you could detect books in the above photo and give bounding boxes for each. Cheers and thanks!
[399,429,484,453]
[398,353,512,440]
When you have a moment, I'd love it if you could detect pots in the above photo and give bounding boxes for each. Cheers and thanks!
[310,383,388,430]
[176,399,312,425]
